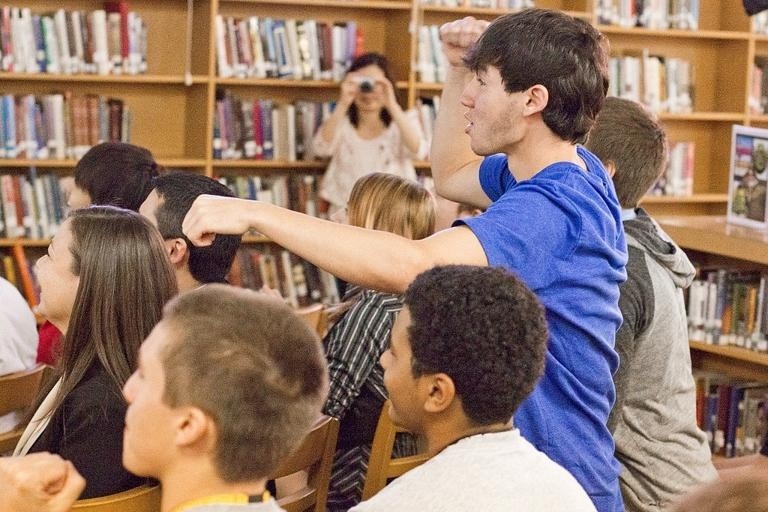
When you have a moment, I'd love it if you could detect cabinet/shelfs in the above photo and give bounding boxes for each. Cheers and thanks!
[417,0,589,176]
[656,218,768,462]
[0,0,215,309]
[749,9,768,122]
[213,0,416,306]
[589,2,754,206]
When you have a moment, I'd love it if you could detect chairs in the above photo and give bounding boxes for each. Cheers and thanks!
[0,366,45,458]
[297,306,326,336]
[250,417,339,512]
[360,398,429,500]
[70,481,162,512]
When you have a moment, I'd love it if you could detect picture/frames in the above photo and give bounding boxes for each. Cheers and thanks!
[726,124,768,231]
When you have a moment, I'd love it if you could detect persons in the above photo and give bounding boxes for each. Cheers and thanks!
[0,9,768,511]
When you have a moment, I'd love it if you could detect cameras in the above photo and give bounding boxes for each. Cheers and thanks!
[352,73,379,92]
